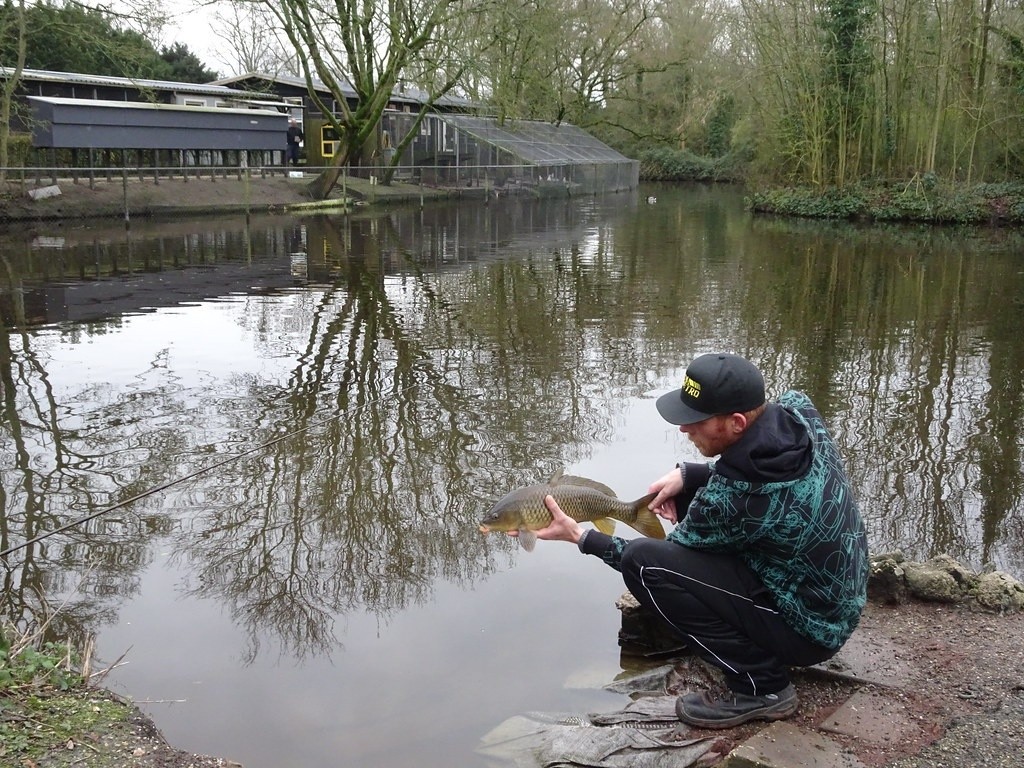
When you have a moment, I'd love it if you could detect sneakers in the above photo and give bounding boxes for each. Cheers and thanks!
[674,677,799,728]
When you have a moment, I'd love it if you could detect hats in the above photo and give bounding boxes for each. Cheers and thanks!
[655,353,765,426]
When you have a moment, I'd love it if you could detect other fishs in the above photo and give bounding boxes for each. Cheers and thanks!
[479,466,667,553]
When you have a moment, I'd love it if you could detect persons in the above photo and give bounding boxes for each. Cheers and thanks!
[503,354,868,729]
[287,118,304,167]
